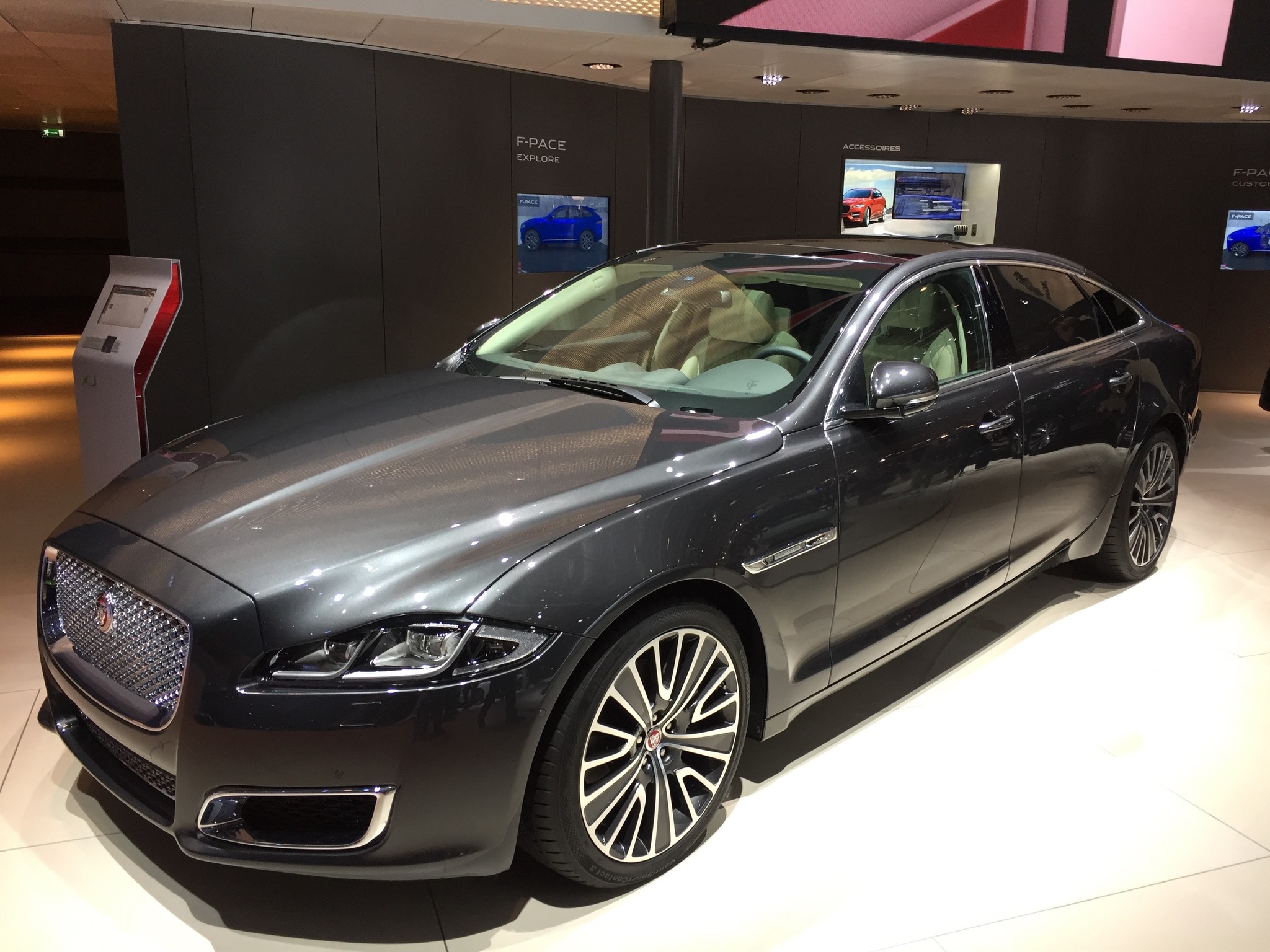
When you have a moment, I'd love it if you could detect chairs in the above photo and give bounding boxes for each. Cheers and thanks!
[680,289,801,380]
[862,284,960,404]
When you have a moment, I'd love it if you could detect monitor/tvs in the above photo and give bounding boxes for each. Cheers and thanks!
[97,285,157,329]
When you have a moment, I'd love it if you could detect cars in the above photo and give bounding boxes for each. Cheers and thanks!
[31,233,1205,900]
[1226,222,1270,258]
[893,176,961,219]
[520,204,603,252]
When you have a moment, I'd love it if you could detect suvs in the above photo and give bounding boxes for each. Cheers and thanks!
[842,188,887,228]
